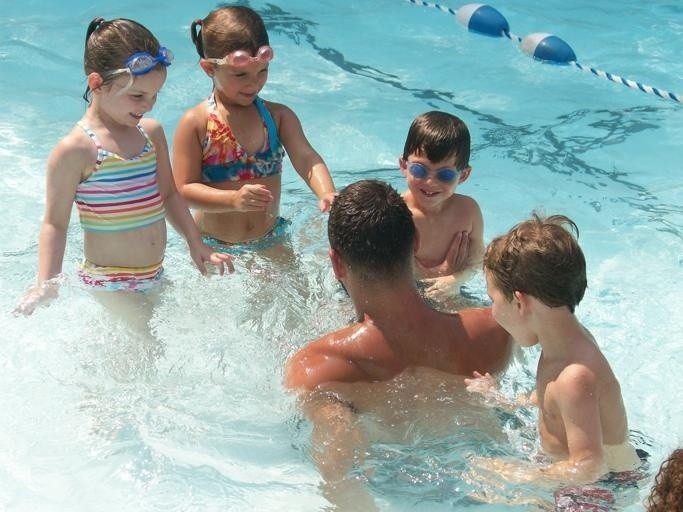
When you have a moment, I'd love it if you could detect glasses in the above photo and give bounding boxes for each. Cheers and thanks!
[98,46,174,81]
[201,46,274,67]
[403,156,467,184]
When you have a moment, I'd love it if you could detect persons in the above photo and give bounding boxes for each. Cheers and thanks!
[170,5,342,339]
[9,16,237,372]
[637,444,683,511]
[276,178,522,511]
[390,109,491,318]
[458,205,649,511]
